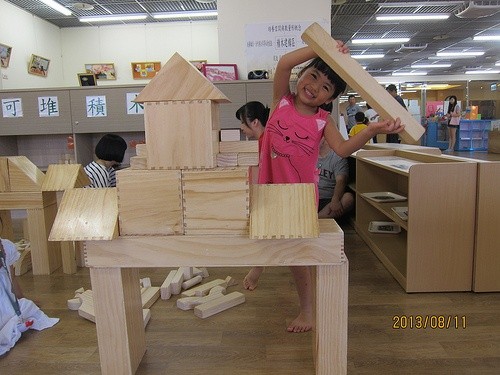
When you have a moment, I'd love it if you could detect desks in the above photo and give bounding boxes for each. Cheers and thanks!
[83,217,350,375]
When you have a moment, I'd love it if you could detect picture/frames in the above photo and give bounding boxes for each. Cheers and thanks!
[85,63,117,80]
[203,64,238,82]
[131,62,162,80]
[28,53,51,78]
[189,60,208,75]
[77,73,98,87]
[0,43,12,68]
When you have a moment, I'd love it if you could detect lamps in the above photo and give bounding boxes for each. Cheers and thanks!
[464,70,500,74]
[391,72,427,76]
[351,37,410,45]
[378,1,464,7]
[435,50,485,57]
[351,54,386,59]
[375,13,451,21]
[472,35,500,42]
[410,64,451,69]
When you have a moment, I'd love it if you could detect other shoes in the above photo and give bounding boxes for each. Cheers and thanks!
[443,148,454,152]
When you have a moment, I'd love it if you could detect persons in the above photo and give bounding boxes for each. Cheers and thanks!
[445,96,461,151]
[237,102,273,160]
[81,133,128,187]
[346,85,408,146]
[303,121,355,219]
[234,40,404,332]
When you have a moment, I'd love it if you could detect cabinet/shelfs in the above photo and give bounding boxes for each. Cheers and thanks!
[346,143,478,294]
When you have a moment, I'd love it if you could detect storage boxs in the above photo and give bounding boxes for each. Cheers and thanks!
[456,119,491,151]
